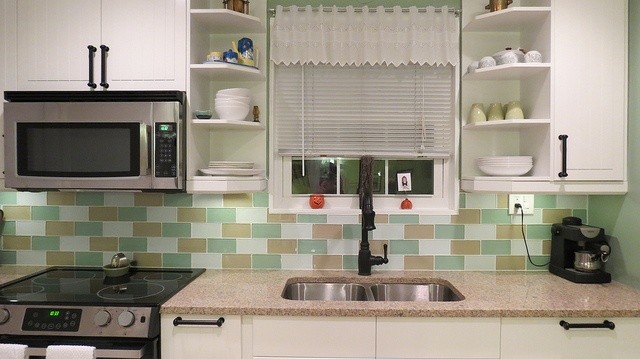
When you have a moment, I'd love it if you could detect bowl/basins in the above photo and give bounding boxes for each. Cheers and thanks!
[215,87,251,119]
[193,110,214,119]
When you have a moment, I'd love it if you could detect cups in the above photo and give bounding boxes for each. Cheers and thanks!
[478,56,496,67]
[523,51,543,62]
[224,49,237,63]
[467,60,479,72]
[500,53,519,64]
[206,51,221,63]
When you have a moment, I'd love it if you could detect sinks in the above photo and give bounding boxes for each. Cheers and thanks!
[280,277,372,302]
[365,279,466,302]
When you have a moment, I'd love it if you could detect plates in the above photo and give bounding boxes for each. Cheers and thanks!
[479,156,533,176]
[198,161,265,175]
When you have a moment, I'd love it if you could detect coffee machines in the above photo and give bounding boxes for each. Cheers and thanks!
[549,217,612,283]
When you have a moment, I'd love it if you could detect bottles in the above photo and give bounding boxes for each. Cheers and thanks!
[488,103,504,121]
[504,102,524,118]
[470,103,487,121]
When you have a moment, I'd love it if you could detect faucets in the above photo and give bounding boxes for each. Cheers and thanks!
[351,192,389,276]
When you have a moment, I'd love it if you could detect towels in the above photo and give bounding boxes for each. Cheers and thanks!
[0,343,28,359]
[46,345,96,359]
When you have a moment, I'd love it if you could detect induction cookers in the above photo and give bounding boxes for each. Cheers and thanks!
[1,266,206,340]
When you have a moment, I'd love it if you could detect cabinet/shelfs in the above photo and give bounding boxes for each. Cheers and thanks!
[1,1,185,93]
[500,315,640,358]
[243,316,501,358]
[549,0,629,195]
[160,314,243,359]
[461,1,550,194]
[185,0,268,193]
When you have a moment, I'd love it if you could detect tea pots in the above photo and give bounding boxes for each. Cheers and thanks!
[231,37,260,66]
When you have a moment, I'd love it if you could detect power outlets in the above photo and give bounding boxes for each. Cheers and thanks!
[508,194,536,216]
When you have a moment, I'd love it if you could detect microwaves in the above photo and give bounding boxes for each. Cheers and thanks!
[3,101,185,192]
